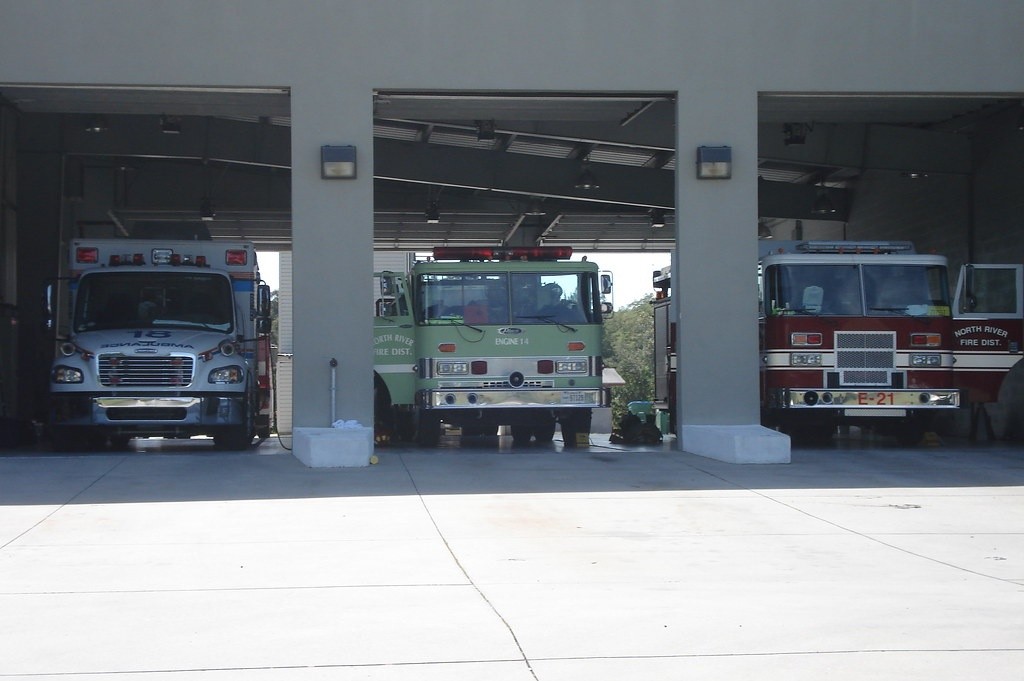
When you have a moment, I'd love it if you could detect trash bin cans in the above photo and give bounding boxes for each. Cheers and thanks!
[627,401,652,423]
[655,411,669,434]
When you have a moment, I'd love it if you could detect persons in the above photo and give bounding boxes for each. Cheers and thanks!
[513,283,586,323]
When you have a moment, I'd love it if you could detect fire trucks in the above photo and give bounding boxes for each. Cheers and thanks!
[373,245,616,454]
[650,240,1024,448]
[38,234,277,451]
